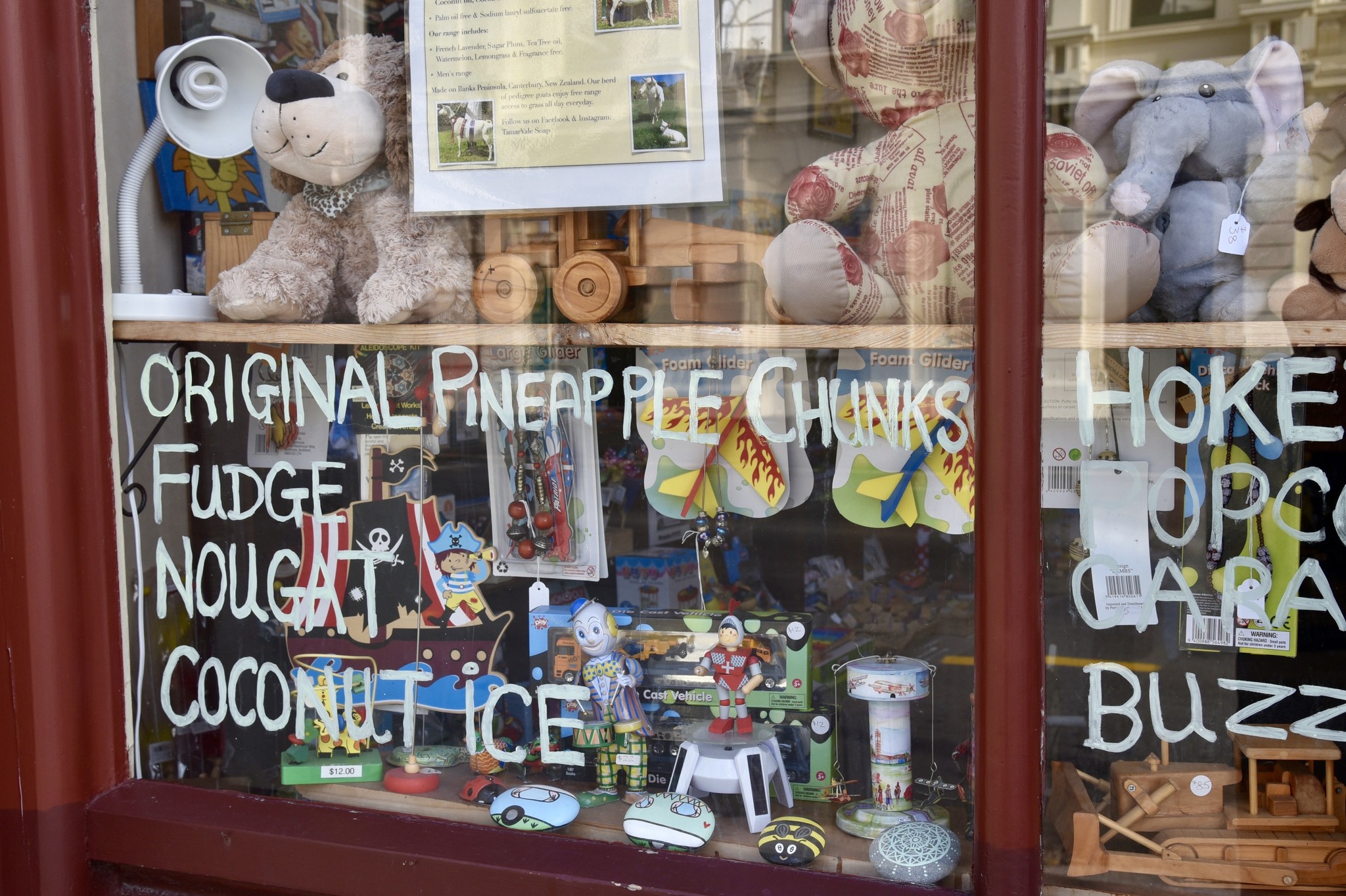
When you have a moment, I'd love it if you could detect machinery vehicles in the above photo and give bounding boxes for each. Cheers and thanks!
[554,631,786,694]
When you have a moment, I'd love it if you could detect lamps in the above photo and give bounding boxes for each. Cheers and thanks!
[112,35,273,321]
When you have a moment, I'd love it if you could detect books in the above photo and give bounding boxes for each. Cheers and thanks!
[139,81,267,295]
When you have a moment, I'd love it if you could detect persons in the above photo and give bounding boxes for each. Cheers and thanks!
[567,597,654,807]
[694,599,763,734]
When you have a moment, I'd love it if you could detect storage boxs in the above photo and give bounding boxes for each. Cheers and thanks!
[528,546,839,804]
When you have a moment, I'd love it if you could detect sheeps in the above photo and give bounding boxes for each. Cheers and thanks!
[438,103,494,162]
[637,75,687,146]
[606,0,656,28]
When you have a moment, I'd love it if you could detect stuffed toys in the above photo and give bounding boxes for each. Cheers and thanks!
[1281,168,1346,323]
[760,0,1161,327]
[1074,37,1305,322]
[208,34,478,326]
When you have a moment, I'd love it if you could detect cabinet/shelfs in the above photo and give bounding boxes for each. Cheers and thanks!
[108,312,1346,896]
